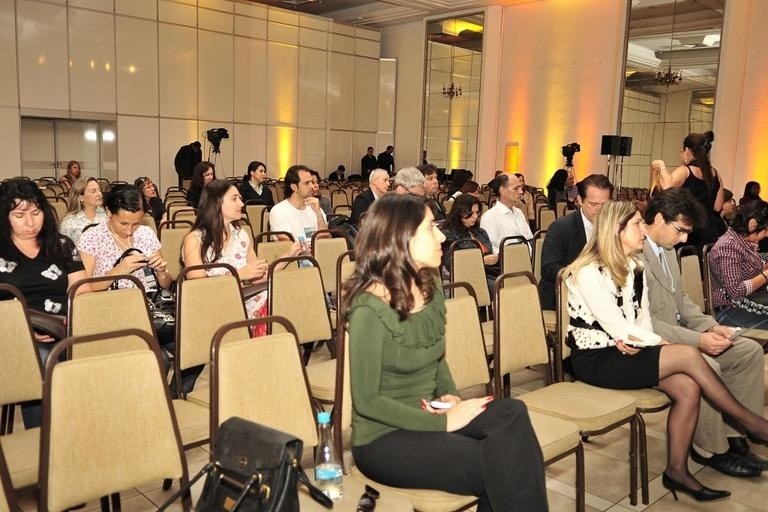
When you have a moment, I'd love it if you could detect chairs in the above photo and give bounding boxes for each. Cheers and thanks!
[0,174,768,511]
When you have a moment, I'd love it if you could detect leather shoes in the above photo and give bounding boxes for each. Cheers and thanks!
[689,446,761,479]
[721,436,766,469]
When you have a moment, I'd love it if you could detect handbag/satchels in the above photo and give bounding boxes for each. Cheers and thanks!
[109,247,179,334]
[195,416,337,512]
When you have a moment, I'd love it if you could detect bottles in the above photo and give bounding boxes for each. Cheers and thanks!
[297,235,316,268]
[314,411,344,502]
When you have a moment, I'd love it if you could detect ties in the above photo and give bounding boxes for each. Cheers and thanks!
[658,249,672,287]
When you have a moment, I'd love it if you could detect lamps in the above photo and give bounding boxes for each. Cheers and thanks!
[600,135,619,186]
[616,136,633,186]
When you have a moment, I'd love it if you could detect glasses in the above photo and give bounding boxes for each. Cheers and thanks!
[355,484,381,512]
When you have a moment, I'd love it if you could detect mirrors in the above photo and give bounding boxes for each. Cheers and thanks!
[614,0,728,191]
[420,10,486,181]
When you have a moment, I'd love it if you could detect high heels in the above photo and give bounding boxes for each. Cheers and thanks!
[662,470,731,502]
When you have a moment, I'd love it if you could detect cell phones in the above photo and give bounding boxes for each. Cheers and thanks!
[431,400,452,409]
[625,343,645,348]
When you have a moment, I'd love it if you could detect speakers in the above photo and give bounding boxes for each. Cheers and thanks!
[601,135,631,154]
